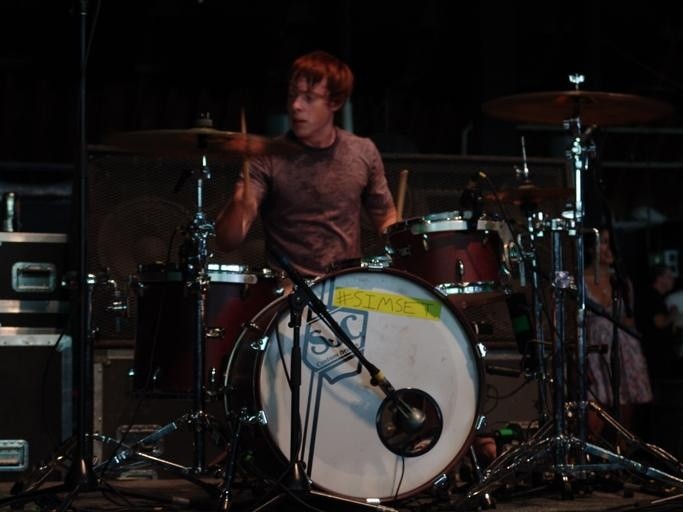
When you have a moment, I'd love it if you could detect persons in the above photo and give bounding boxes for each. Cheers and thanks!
[215,45,414,297]
[642,263,678,397]
[575,226,645,455]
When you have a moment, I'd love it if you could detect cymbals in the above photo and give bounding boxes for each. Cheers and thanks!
[478,92,679,127]
[480,182,573,205]
[115,129,293,158]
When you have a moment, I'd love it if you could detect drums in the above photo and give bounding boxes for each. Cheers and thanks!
[130,263,281,401]
[382,212,514,310]
[223,257,484,504]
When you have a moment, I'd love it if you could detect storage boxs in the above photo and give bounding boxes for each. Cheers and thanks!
[0,329,74,483]
[0,232,71,315]
